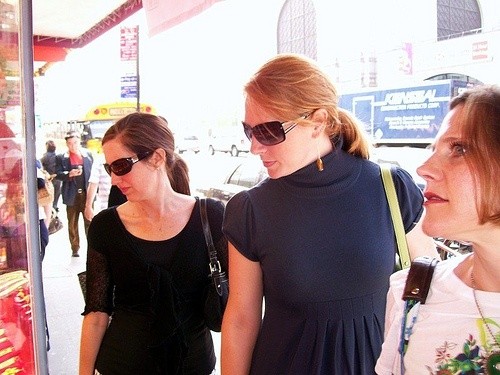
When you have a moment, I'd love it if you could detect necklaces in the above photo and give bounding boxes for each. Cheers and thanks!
[471,270,500,346]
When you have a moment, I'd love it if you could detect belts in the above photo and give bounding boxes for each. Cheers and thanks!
[75,188,85,194]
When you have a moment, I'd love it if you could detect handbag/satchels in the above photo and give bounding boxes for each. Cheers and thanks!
[77,271,115,317]
[200,198,229,333]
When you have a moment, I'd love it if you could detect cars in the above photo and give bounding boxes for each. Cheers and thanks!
[175,126,253,158]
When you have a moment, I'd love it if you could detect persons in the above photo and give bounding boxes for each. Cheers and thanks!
[84,152,113,221]
[374,84,500,375]
[41,140,61,213]
[79,113,229,375]
[107,116,168,208]
[220,56,441,375]
[0,138,54,352]
[54,130,99,257]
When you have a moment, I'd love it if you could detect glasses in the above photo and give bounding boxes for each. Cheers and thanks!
[242,112,312,146]
[104,151,153,177]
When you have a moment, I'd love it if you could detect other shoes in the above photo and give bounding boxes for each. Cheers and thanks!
[72,252,79,257]
